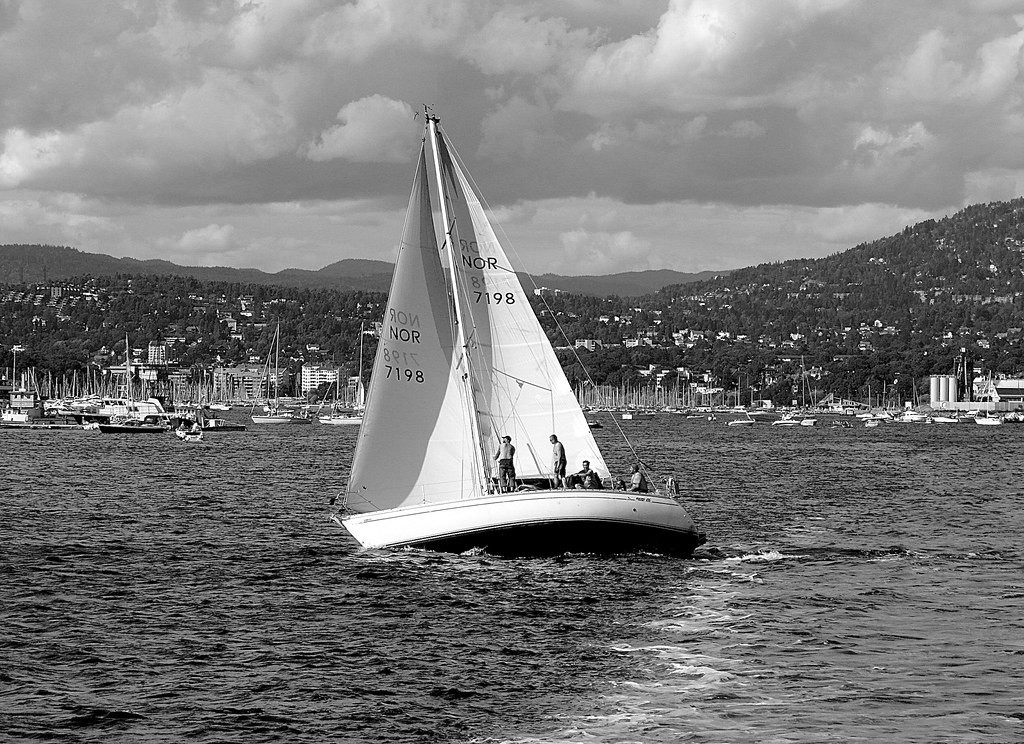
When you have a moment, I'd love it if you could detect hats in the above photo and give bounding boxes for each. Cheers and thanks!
[502,436,511,441]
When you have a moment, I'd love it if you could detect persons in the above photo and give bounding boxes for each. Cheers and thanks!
[178,421,185,430]
[614,475,625,490]
[550,435,569,489]
[626,463,648,492]
[191,421,198,432]
[573,461,598,489]
[493,436,516,494]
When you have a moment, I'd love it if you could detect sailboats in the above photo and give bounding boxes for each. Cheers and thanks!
[315,321,365,425]
[973,370,1004,426]
[0,333,247,433]
[249,316,313,423]
[329,103,708,559]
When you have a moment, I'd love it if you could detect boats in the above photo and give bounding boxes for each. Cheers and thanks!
[746,407,776,422]
[725,420,755,427]
[772,355,985,427]
[622,409,655,420]
[175,428,204,442]
